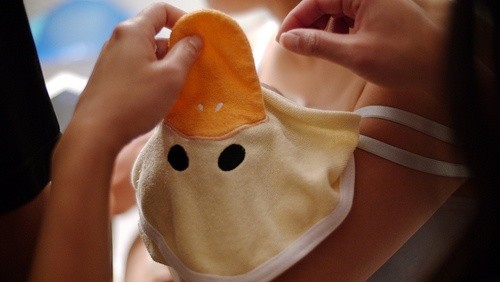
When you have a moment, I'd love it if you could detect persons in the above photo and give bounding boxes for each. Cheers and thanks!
[0,0,60,282]
[122,0,469,279]
[31,0,500,279]
